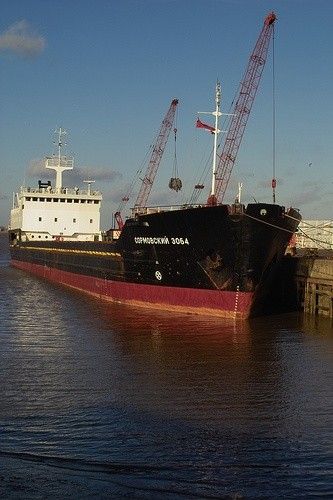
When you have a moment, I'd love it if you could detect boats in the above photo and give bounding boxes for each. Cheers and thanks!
[8,10,302,321]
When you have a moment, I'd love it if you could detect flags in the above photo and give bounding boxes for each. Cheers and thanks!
[195,119,215,134]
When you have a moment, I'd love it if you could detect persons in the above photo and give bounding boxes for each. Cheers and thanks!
[289,234,295,247]
